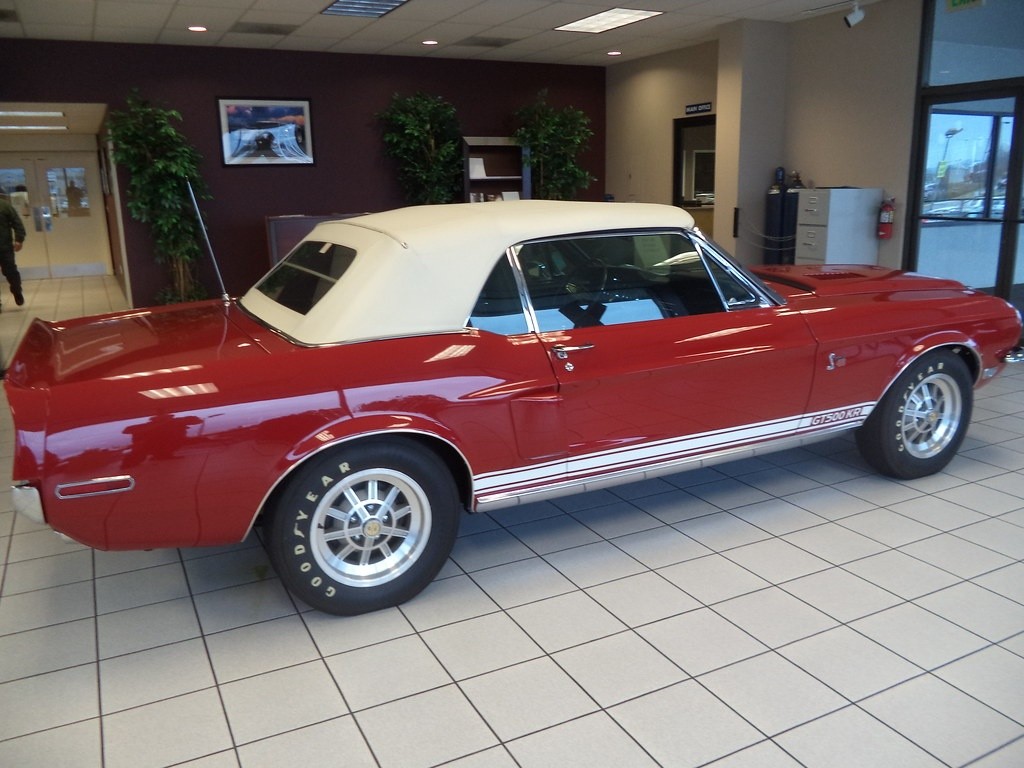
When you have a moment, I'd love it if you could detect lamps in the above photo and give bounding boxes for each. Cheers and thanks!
[844,0,864,27]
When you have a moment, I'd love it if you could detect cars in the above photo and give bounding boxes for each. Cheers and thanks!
[4,198,1023,616]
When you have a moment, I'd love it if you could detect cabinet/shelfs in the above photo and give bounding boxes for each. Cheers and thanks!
[794,188,883,265]
[461,137,530,203]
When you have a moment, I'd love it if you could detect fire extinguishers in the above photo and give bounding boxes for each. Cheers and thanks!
[877,196,896,239]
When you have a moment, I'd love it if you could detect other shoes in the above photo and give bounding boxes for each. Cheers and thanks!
[13,293,24,305]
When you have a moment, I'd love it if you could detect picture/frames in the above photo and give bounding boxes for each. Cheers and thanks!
[216,96,316,167]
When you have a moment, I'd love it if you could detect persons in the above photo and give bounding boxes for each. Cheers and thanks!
[10,184,30,217]
[0,198,26,313]
[66,180,83,209]
